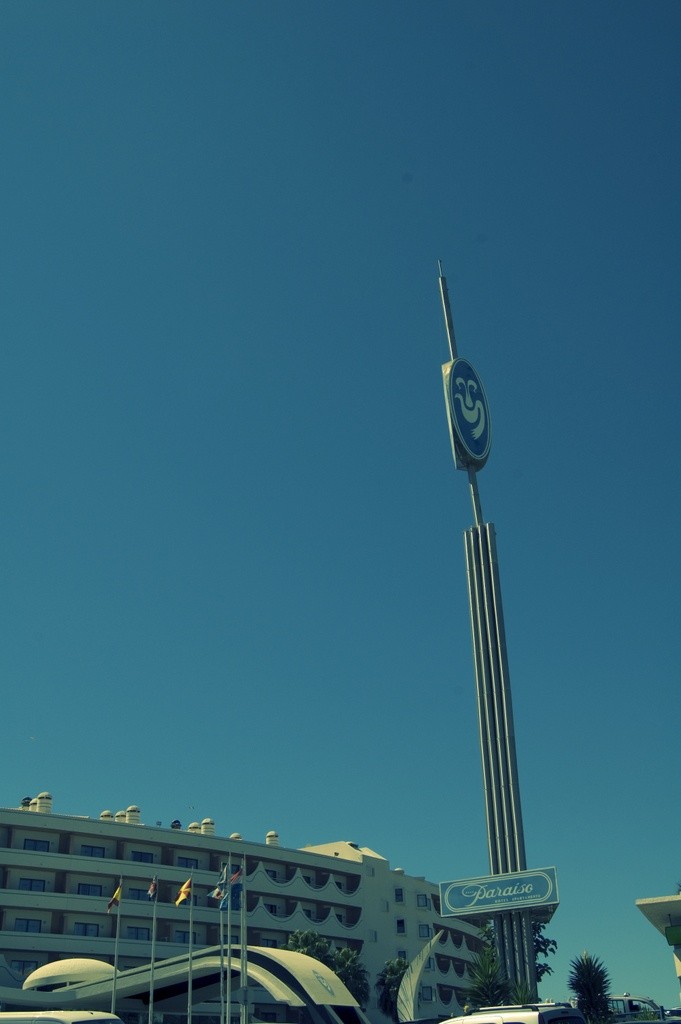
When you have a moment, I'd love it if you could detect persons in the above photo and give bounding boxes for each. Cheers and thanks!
[463,1006,472,1016]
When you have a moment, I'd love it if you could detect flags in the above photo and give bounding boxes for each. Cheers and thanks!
[175,877,191,907]
[147,874,157,902]
[206,864,242,911]
[108,884,122,914]
